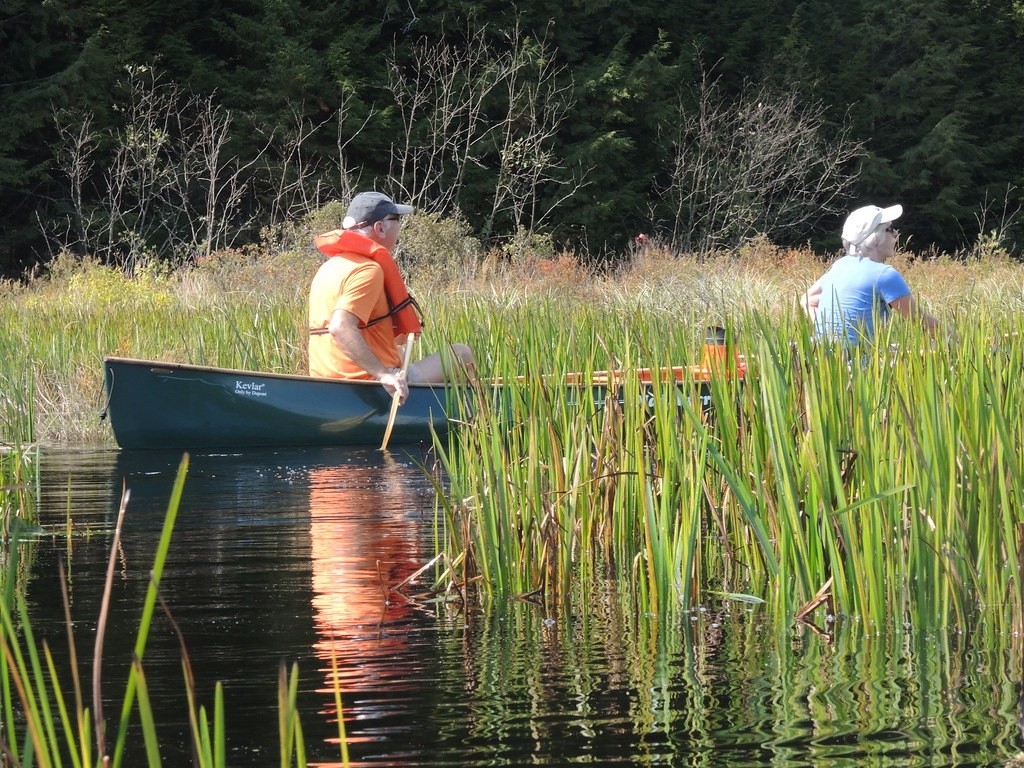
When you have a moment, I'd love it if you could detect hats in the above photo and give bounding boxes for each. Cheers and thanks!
[841,205,903,254]
[342,192,414,230]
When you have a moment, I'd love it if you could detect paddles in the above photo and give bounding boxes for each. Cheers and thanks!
[382,331,417,451]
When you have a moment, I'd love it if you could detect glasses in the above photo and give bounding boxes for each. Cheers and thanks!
[886,224,893,233]
[372,214,399,228]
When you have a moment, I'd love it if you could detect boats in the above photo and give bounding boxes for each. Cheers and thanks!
[103,355,1003,447]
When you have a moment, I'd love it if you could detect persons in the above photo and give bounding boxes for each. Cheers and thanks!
[800,204,958,361]
[309,192,474,407]
